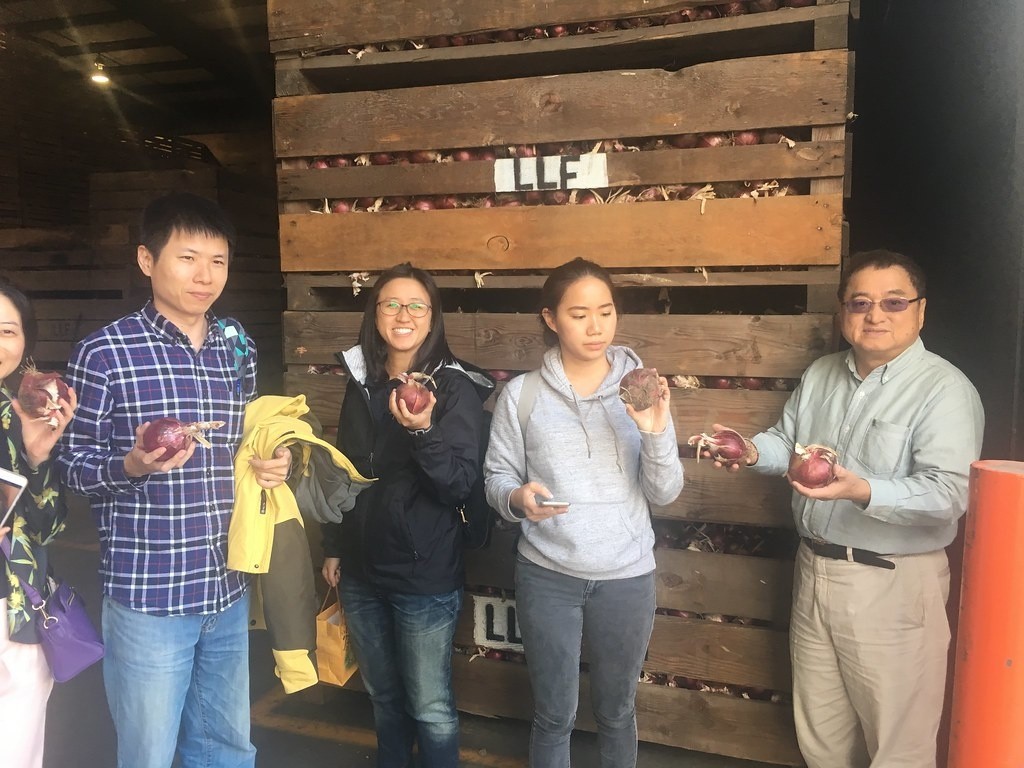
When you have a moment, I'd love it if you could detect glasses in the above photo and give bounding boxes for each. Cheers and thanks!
[841,296,927,313]
[376,301,432,318]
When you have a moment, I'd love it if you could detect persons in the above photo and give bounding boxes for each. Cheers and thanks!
[0,277,78,768]
[482,259,685,768]
[323,260,495,768]
[49,191,294,768]
[701,248,984,768]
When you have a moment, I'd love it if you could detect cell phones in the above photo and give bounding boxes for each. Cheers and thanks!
[538,501,571,509]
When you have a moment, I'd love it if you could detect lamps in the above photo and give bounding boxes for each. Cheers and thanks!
[89,54,109,89]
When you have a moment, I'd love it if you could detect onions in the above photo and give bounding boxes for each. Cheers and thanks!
[304,0,815,700]
[786,443,838,489]
[143,417,226,463]
[18,355,69,421]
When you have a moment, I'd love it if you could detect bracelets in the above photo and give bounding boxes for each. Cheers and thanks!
[408,427,434,435]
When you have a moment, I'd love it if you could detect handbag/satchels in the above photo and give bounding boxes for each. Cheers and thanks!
[315,583,359,687]
[36,575,105,683]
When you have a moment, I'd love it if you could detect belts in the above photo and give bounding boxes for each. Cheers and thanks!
[802,537,895,569]
